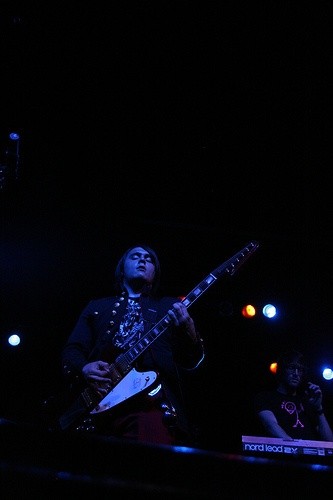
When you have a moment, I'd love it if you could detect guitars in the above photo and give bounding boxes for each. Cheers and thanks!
[79,240,259,415]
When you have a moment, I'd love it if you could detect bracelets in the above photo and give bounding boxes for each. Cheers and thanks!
[310,409,324,415]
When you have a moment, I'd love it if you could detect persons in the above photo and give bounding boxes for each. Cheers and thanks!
[58,244,209,447]
[246,350,332,442]
